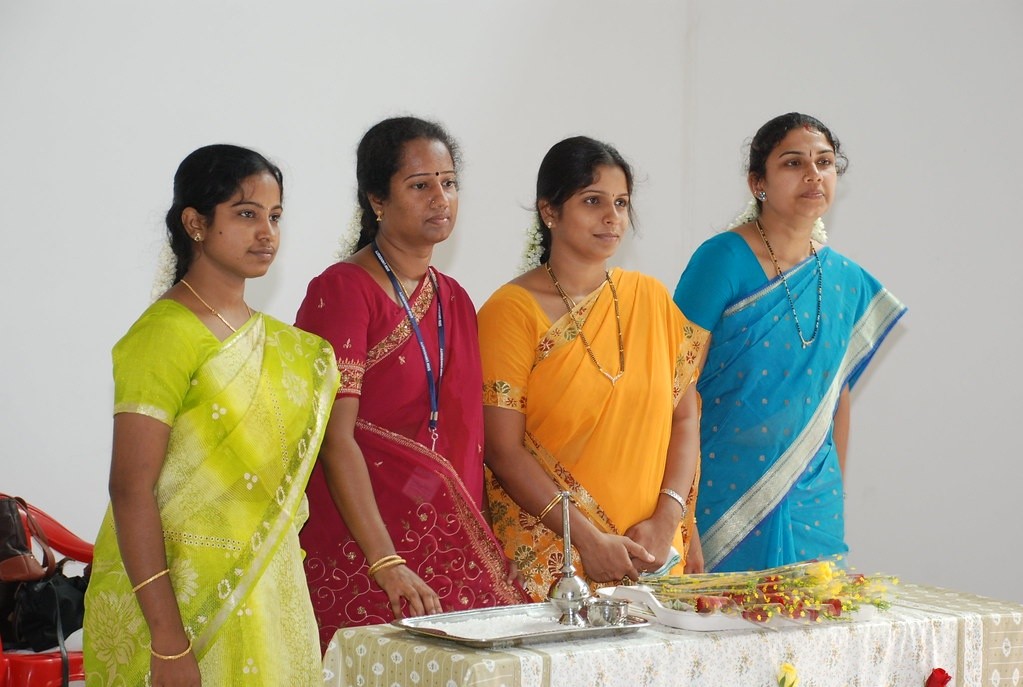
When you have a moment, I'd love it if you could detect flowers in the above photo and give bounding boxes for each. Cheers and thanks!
[637,550,902,627]
[775,661,800,687]
[923,668,952,687]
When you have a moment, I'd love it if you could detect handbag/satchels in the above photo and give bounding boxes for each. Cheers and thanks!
[0,555,93,652]
[0,497,55,582]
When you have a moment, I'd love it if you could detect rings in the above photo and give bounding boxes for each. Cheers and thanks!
[643,569,647,572]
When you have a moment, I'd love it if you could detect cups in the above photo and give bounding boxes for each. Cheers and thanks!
[594,600,632,628]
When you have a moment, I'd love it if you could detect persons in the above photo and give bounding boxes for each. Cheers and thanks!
[669,110,908,573]
[290,115,534,662]
[83,142,340,687]
[476,133,713,602]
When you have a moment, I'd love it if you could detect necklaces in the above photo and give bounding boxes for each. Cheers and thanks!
[541,259,625,388]
[753,217,823,347]
[179,278,253,333]
[383,256,411,301]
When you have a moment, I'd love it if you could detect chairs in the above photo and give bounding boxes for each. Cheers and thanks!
[0,494,95,687]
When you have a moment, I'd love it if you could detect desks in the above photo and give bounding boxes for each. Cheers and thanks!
[322,581,1023,687]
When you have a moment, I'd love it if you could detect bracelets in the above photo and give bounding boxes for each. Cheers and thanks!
[535,495,562,524]
[366,554,406,578]
[148,637,193,661]
[129,566,170,594]
[659,489,687,517]
